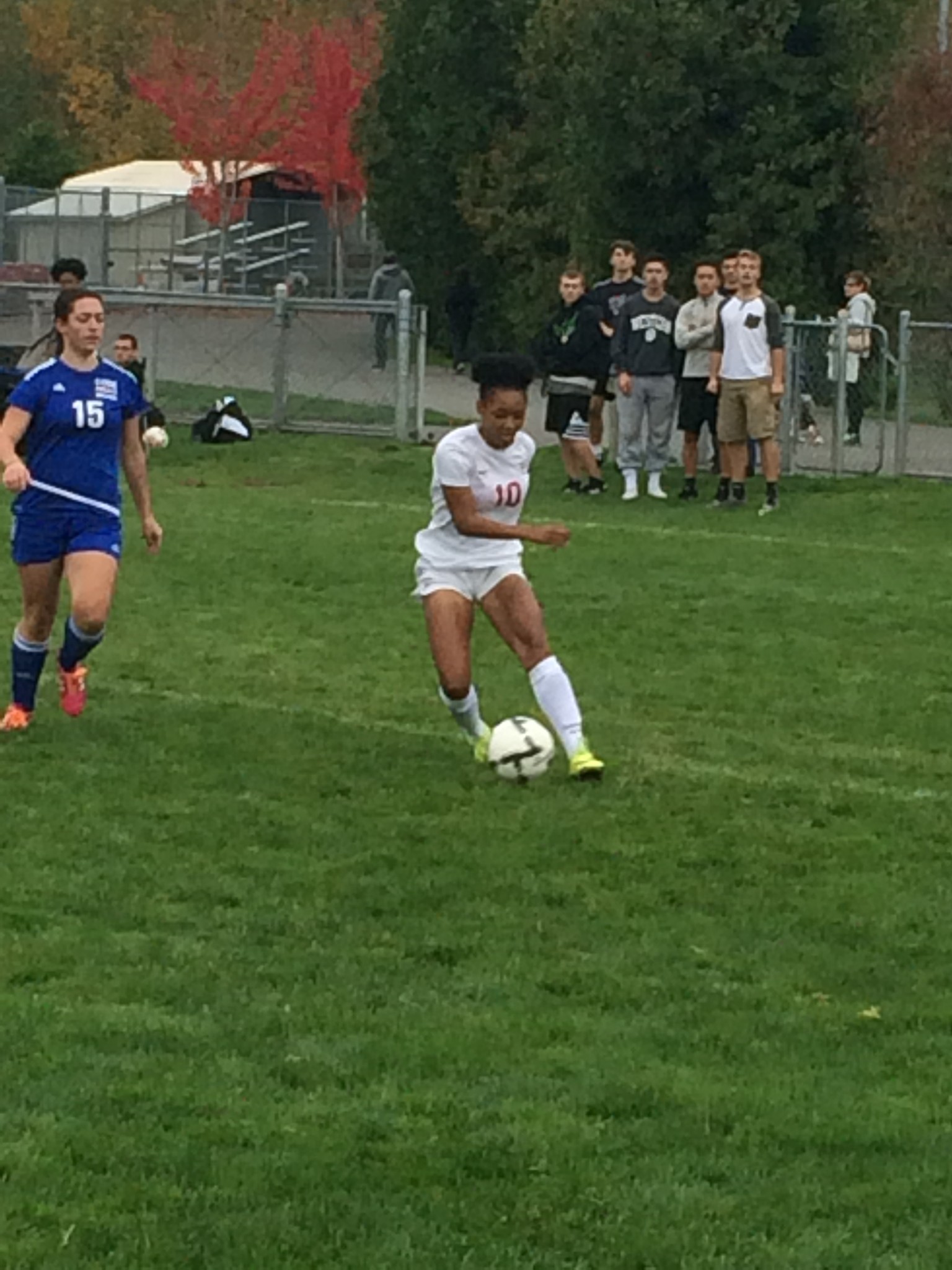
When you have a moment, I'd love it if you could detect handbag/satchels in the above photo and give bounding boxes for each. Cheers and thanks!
[828,351,860,383]
[828,298,872,350]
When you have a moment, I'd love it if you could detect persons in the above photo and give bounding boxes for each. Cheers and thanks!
[112,333,144,396]
[409,353,606,779]
[0,288,163,734]
[790,378,824,446]
[283,266,308,316]
[824,271,877,449]
[367,249,415,372]
[535,238,783,513]
[27,257,89,363]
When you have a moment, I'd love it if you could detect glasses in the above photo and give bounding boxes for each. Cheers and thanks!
[844,282,860,288]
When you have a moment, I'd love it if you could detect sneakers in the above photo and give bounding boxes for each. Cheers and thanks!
[55,647,87,717]
[0,704,30,730]
[568,752,602,778]
[470,726,491,762]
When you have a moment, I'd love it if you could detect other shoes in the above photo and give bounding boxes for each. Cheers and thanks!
[584,478,608,495]
[844,433,859,445]
[562,478,580,494]
[759,504,776,516]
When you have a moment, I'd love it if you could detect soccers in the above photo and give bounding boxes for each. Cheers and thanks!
[142,425,169,448]
[486,715,556,783]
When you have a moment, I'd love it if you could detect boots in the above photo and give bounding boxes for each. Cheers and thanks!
[646,473,668,498]
[622,470,639,498]
